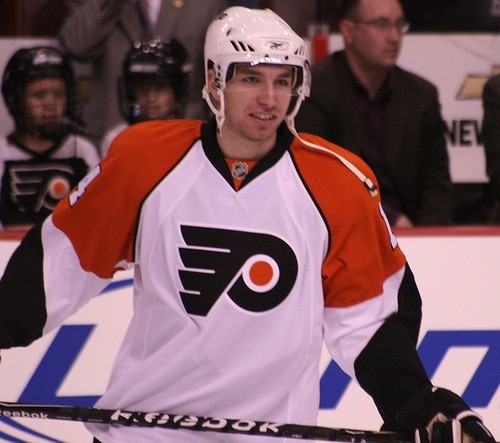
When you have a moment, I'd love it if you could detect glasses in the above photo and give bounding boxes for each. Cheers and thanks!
[351,18,410,35]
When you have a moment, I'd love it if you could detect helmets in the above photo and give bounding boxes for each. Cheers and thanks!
[2,45,78,135]
[114,37,191,126]
[201,6,310,119]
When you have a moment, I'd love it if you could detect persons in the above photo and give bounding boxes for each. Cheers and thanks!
[100,33,195,162]
[479,70,499,236]
[1,5,498,443]
[287,0,453,231]
[0,45,104,243]
[59,0,267,130]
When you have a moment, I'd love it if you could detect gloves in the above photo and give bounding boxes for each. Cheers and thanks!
[380,384,496,443]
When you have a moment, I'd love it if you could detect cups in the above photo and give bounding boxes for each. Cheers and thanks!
[307,23,330,62]
